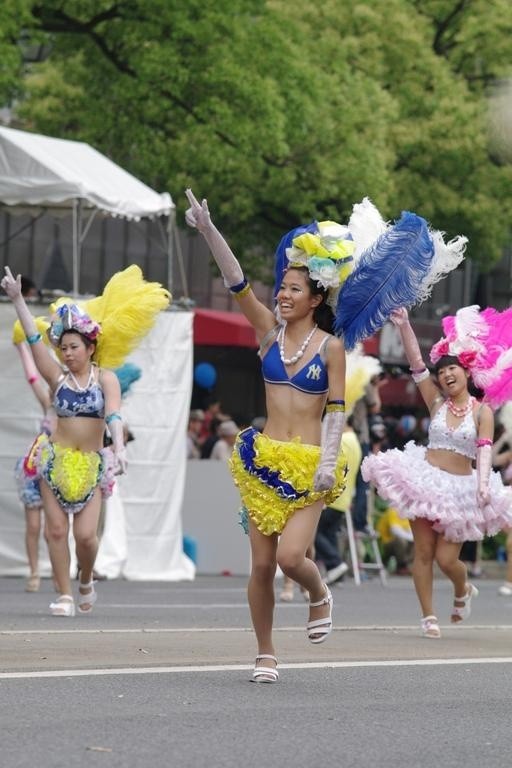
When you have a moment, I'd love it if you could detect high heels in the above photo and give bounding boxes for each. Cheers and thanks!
[450,582,479,624]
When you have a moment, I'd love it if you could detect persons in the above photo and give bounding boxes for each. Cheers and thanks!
[187,375,512,583]
[362,312,512,638]
[184,190,468,683]
[1,266,173,617]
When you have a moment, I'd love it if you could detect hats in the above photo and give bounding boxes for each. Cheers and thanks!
[216,420,239,439]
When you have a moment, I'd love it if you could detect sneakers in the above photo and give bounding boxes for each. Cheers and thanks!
[307,583,334,643]
[421,615,441,639]
[50,595,76,617]
[24,571,41,591]
[77,570,97,614]
[279,585,295,600]
[253,654,280,683]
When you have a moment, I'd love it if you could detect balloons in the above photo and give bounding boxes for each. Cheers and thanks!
[196,363,215,385]
